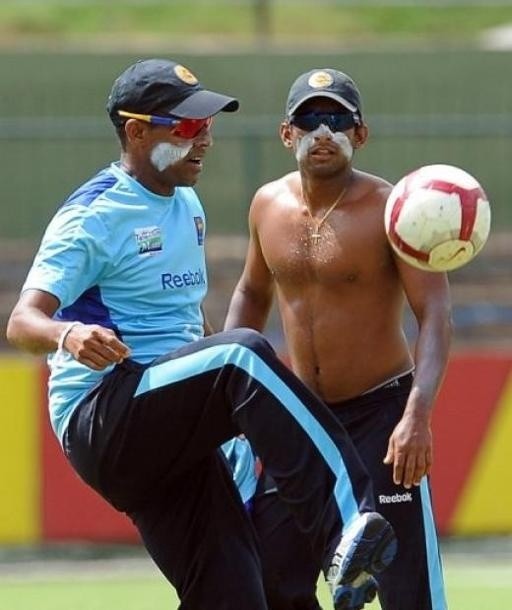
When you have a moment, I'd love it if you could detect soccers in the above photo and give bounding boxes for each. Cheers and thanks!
[384,164,491,272]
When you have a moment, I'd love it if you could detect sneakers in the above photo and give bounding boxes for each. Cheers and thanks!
[327,512,398,610]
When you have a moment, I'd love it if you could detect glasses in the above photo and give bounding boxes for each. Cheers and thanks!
[117,110,211,138]
[289,113,360,132]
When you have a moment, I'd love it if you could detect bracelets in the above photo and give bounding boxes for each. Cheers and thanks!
[58,319,83,361]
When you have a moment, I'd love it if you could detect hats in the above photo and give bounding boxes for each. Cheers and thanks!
[107,59,238,127]
[287,69,362,115]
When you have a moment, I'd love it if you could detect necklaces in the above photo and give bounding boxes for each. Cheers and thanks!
[299,184,346,244]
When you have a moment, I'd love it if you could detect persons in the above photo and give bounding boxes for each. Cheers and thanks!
[223,68,448,609]
[6,59,397,610]
